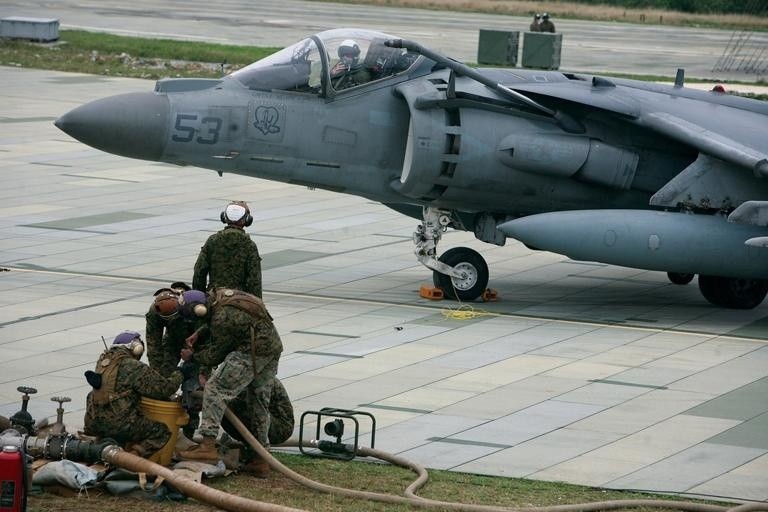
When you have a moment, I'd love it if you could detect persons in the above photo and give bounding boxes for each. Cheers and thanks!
[530,12,555,32]
[192,201,262,298]
[330,39,370,91]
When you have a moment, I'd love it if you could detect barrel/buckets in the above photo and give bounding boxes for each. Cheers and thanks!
[0,445,29,512]
[125,396,189,467]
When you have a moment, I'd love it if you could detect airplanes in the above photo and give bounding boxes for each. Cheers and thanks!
[48,23,768,309]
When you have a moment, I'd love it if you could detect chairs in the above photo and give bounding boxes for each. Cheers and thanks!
[361,36,402,80]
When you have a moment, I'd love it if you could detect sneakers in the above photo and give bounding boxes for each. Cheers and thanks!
[176,436,218,465]
[243,458,270,478]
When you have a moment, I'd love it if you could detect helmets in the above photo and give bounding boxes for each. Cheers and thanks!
[338,38,361,57]
[221,201,253,228]
[111,330,145,360]
[153,288,208,319]
